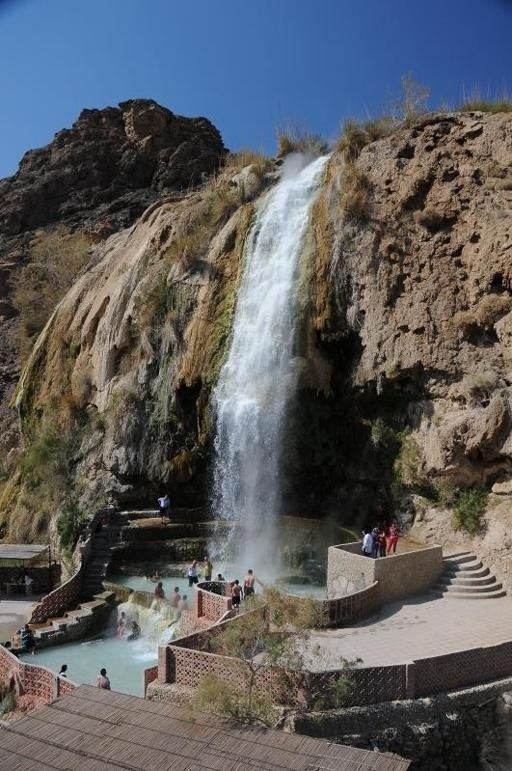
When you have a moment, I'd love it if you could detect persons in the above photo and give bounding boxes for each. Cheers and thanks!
[171,587,181,608]
[370,528,385,559]
[217,574,226,582]
[95,668,112,691]
[229,583,235,595]
[387,522,399,555]
[32,647,41,657]
[177,595,188,611]
[117,612,127,639]
[232,580,243,613]
[361,529,373,557]
[243,569,265,600]
[377,529,387,557]
[126,621,141,641]
[154,582,165,598]
[58,665,67,679]
[203,556,213,582]
[188,560,202,588]
[157,493,172,529]
